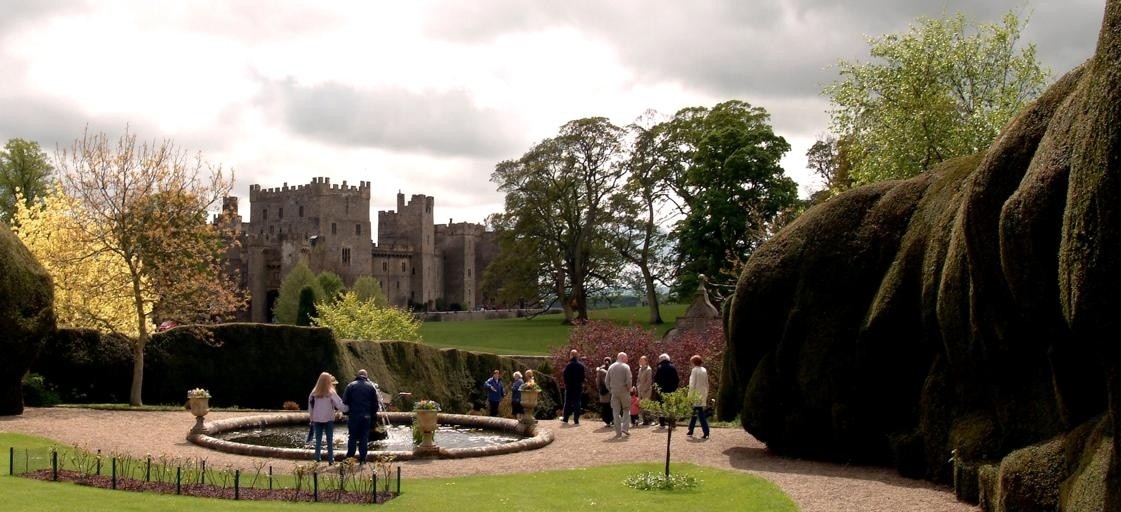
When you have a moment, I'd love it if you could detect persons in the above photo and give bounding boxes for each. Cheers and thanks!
[307,371,351,465]
[637,354,653,404]
[557,347,586,425]
[654,353,680,431]
[628,386,643,427]
[594,356,615,427]
[509,370,526,421]
[685,354,710,439]
[343,368,380,464]
[605,350,634,440]
[522,368,536,383]
[483,368,506,414]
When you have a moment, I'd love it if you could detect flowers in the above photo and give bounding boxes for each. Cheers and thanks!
[520,379,542,393]
[414,399,443,411]
[188,386,211,397]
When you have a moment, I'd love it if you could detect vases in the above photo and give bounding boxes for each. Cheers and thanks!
[518,388,541,423]
[416,411,440,452]
[189,397,210,433]
